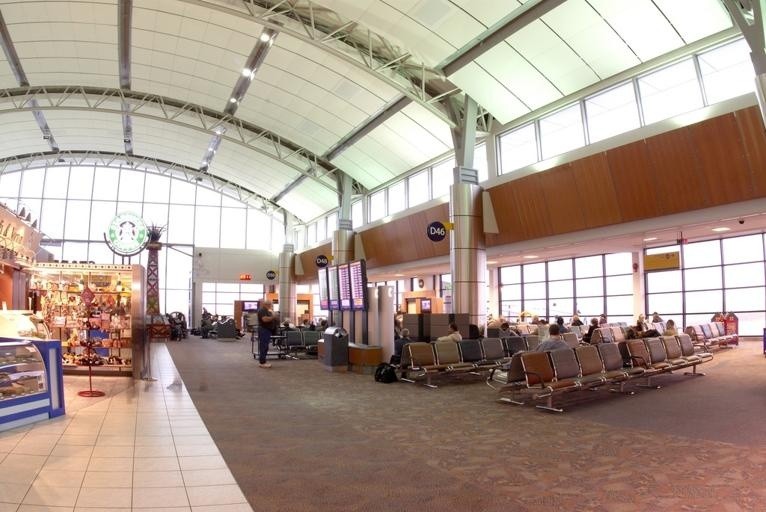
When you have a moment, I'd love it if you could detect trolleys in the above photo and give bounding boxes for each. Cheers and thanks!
[248,323,288,361]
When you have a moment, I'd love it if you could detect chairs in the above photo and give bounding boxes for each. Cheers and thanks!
[149,311,187,341]
[271,326,325,360]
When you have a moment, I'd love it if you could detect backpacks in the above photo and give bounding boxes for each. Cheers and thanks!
[190,328,202,336]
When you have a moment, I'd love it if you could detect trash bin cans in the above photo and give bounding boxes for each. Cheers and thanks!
[324,326,348,373]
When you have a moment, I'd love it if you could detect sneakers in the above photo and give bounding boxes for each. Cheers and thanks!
[258,362,272,368]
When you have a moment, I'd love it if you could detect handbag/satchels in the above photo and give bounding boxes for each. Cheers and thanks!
[374,360,399,385]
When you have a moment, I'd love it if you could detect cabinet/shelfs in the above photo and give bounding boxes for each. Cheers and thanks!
[30,262,146,379]
[0,341,51,432]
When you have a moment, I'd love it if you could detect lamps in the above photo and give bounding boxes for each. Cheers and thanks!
[5,198,38,230]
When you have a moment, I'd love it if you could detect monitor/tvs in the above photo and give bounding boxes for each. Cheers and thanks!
[243,301,260,311]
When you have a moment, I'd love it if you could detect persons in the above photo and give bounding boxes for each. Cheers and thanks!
[257,299,276,368]
[279,315,328,334]
[498,311,679,353]
[398,328,415,358]
[434,322,463,372]
[166,312,187,343]
[200,307,245,341]
